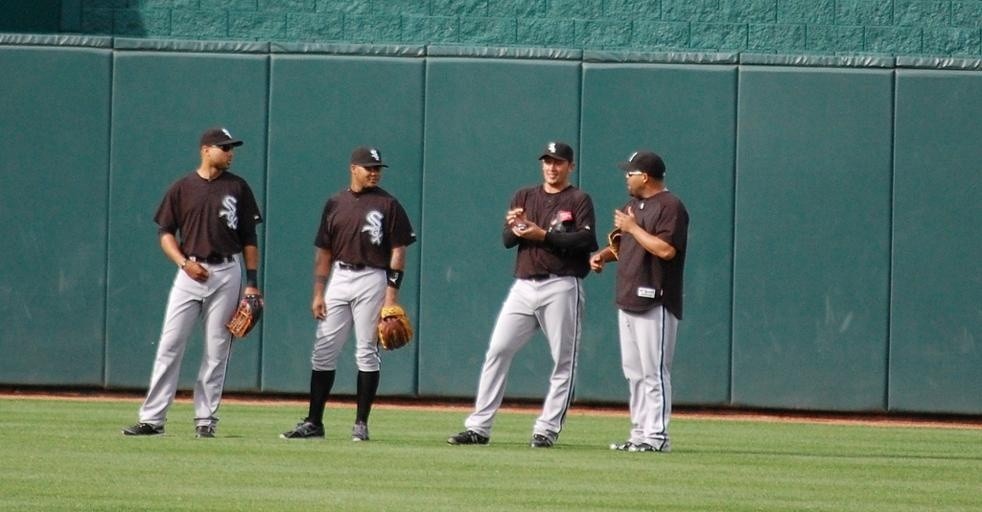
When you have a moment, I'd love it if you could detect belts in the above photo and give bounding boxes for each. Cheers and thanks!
[339,263,365,271]
[185,255,235,263]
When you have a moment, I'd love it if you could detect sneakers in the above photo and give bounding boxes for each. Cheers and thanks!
[352,421,368,441]
[446,430,489,446]
[279,417,325,440]
[196,426,215,437]
[529,434,553,448]
[617,441,658,451]
[122,422,164,435]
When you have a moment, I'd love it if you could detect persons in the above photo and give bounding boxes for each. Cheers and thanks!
[446,140,596,448]
[279,146,417,444]
[588,150,688,452]
[124,125,264,440]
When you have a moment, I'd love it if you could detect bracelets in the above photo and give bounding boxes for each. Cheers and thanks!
[385,265,404,289]
[246,269,258,288]
[180,258,188,269]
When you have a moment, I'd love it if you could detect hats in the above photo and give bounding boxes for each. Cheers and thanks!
[618,152,666,176]
[201,128,244,146]
[352,147,389,168]
[539,142,574,161]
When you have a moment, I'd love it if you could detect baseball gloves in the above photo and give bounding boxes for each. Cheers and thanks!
[225,289,264,338]
[607,227,622,259]
[378,305,412,350]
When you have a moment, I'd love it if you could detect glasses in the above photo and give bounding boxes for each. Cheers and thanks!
[211,145,233,152]
[626,171,643,176]
